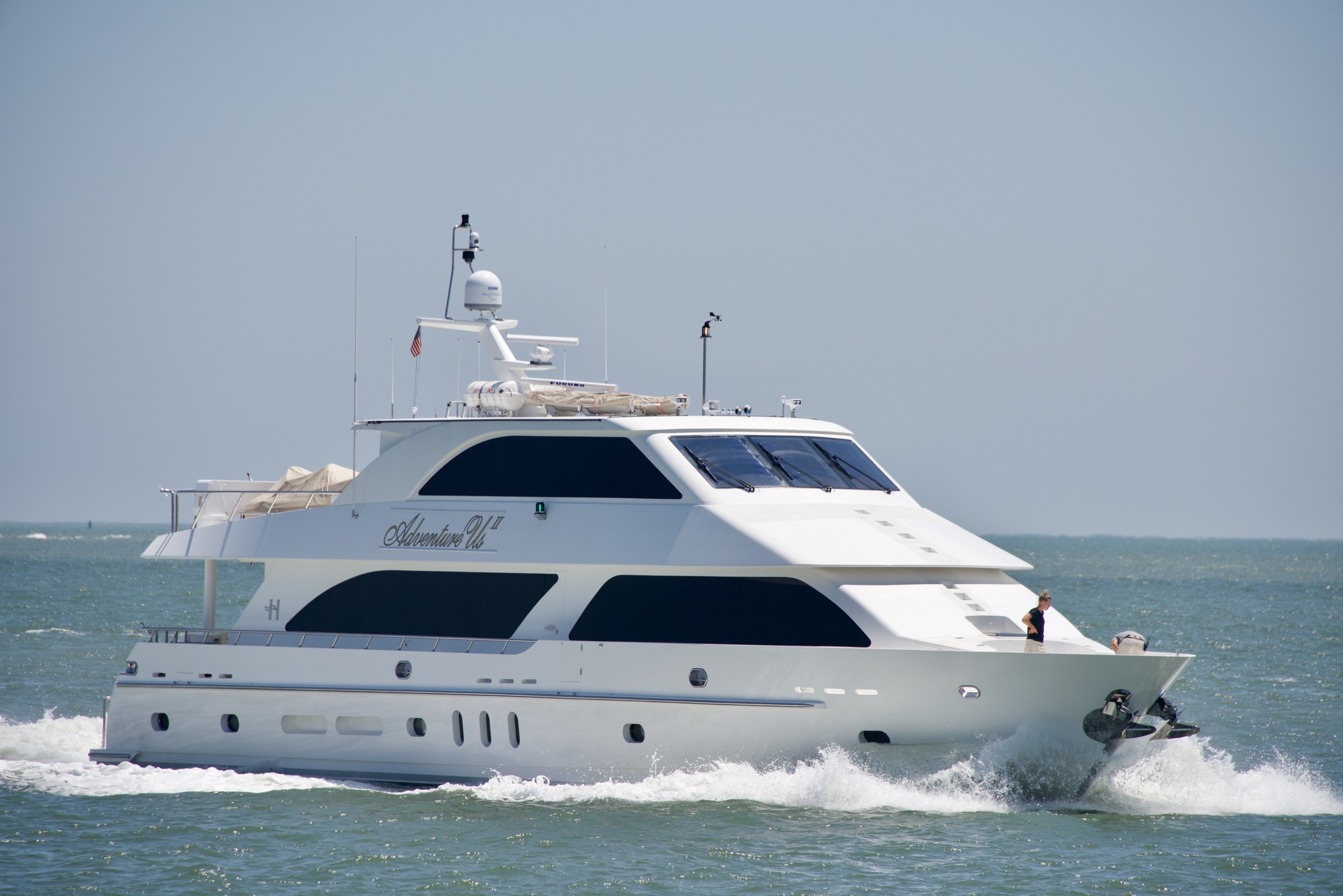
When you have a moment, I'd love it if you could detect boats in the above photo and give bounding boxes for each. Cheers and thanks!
[89,214,1196,800]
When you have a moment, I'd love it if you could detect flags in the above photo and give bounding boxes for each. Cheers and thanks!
[411,326,422,357]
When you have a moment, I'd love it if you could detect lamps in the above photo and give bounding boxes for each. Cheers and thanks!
[702,325,711,336]
[785,399,801,410]
[671,397,687,407]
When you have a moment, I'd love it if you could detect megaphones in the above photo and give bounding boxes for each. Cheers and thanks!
[702,400,721,412]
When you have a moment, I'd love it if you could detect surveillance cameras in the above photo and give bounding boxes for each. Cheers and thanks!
[463,251,475,264]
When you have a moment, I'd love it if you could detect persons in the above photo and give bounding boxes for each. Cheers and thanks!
[1112,631,1147,654]
[1022,589,1051,652]
